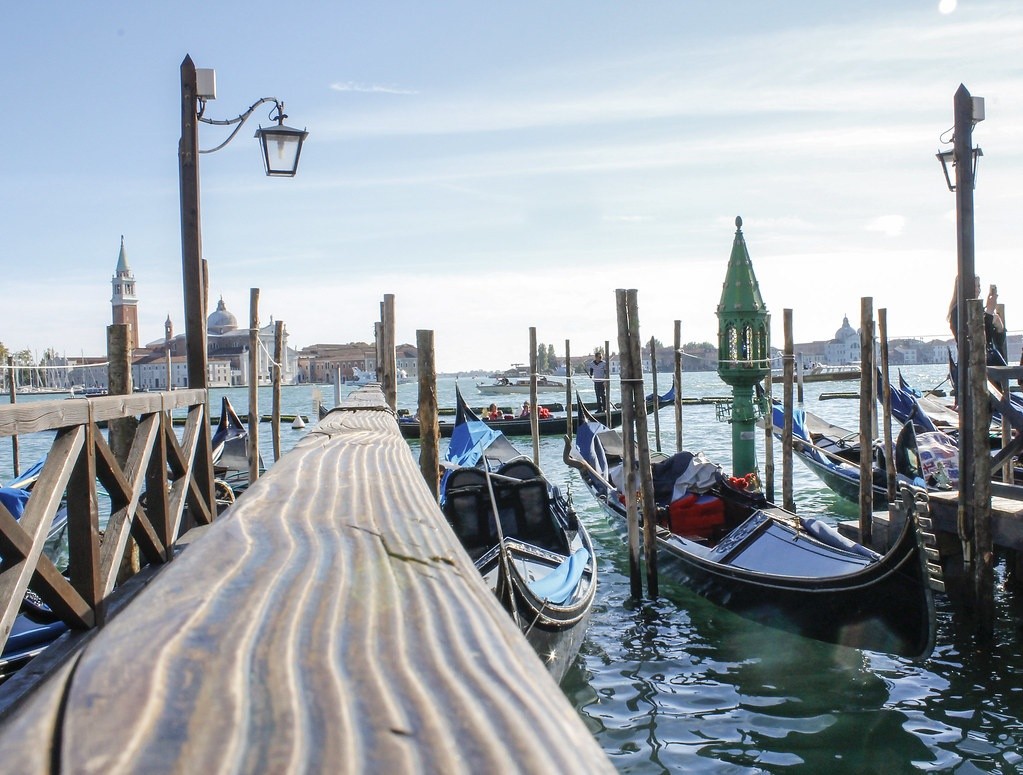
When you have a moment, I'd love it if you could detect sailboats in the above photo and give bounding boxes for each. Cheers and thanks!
[0,347,107,394]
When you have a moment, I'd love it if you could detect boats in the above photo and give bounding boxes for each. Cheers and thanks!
[748,343,1023,555]
[563,387,947,660]
[440,381,597,689]
[85,392,109,398]
[345,367,408,387]
[475,375,578,394]
[396,375,678,439]
[771,365,862,383]
[0,450,68,570]
[211,395,268,516]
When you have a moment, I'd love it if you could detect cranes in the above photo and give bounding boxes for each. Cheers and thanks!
[511,364,524,368]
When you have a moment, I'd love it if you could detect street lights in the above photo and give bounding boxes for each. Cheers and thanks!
[178,53,309,387]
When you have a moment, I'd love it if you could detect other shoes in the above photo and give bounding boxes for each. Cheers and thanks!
[595,409,606,413]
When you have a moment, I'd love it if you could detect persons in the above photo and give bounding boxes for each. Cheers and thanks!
[948,274,1023,467]
[489,403,505,420]
[521,401,530,416]
[804,361,814,369]
[589,352,607,413]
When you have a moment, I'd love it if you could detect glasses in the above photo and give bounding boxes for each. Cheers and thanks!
[597,357,601,358]
